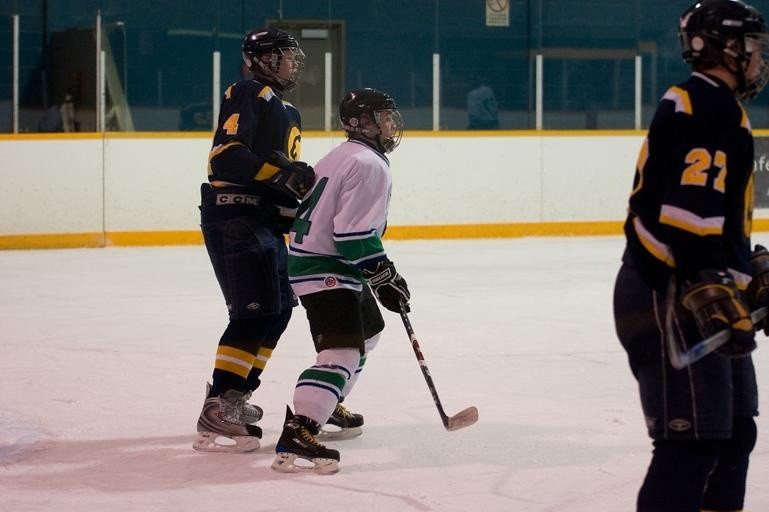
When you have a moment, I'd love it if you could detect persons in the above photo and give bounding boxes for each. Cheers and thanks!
[193,26,316,452]
[614,0,769,510]
[468,74,498,129]
[270,86,411,471]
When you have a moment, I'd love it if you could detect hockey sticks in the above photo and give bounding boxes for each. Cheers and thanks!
[666,275,766,370]
[397,298,479,431]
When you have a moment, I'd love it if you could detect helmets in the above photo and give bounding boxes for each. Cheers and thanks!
[338,87,403,155]
[678,2,768,103]
[241,27,306,96]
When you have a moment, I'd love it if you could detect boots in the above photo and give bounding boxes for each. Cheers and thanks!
[275,406,341,461]
[325,396,363,428]
[196,381,262,439]
[237,389,263,422]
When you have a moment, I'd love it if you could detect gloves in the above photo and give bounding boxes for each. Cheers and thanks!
[266,162,316,202]
[744,244,769,340]
[678,272,757,359]
[361,258,409,314]
